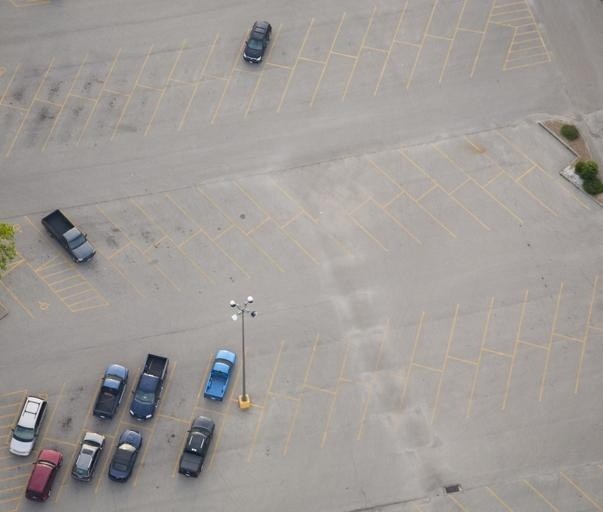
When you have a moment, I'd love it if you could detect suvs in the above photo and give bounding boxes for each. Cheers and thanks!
[8,396,48,458]
[24,448,62,502]
[70,431,105,481]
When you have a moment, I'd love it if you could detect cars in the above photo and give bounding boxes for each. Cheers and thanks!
[242,21,271,64]
[108,429,142,482]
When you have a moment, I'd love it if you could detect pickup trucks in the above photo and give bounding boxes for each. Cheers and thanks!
[40,208,95,263]
[177,415,215,479]
[93,364,129,419]
[203,349,236,401]
[128,352,169,420]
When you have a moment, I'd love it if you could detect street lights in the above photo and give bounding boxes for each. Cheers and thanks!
[229,295,257,411]
